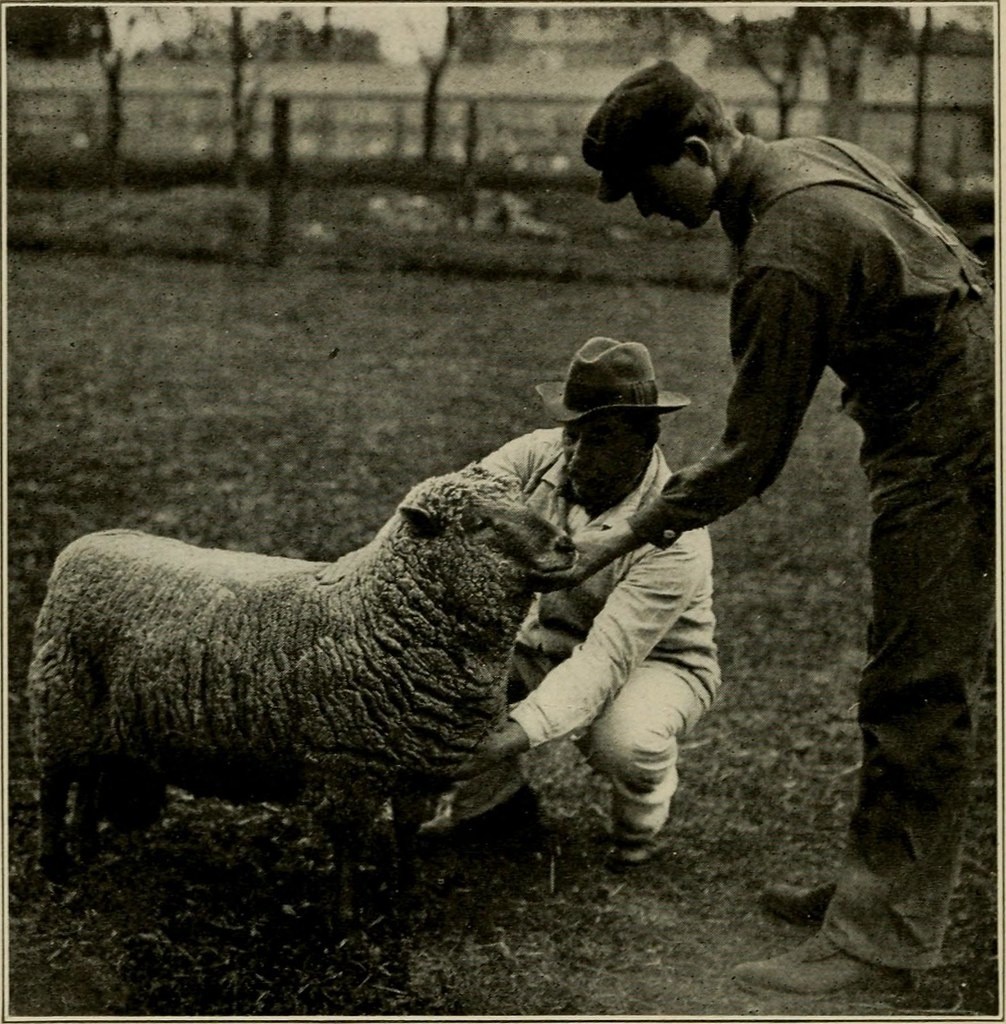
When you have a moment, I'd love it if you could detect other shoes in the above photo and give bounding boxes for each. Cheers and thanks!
[618,839,652,864]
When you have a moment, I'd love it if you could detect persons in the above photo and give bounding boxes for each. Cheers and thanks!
[414,335,722,865]
[520,59,994,1003]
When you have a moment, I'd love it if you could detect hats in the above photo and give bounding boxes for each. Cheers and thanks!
[536,337,691,421]
[582,60,705,203]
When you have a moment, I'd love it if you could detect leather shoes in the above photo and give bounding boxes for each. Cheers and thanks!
[734,930,921,1000]
[760,877,836,925]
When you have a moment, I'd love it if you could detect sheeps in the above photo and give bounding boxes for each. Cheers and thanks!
[24,459,581,909]
